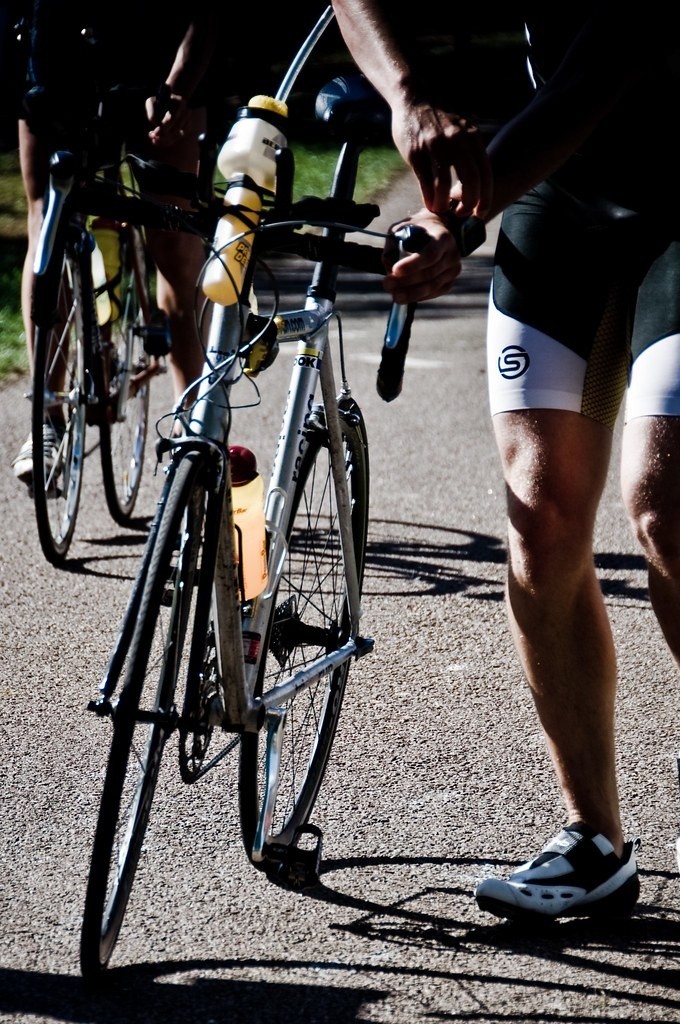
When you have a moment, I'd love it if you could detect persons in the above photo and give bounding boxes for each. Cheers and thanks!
[16,0,274,493]
[329,0,680,918]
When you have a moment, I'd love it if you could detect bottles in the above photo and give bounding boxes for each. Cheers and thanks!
[227,445,268,597]
[201,94,288,305]
[88,232,111,326]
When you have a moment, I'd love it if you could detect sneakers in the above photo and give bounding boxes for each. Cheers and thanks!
[476,822,640,922]
[15,416,66,499]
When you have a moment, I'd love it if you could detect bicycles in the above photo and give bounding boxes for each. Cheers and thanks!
[0,5,204,567]
[75,2,432,992]
